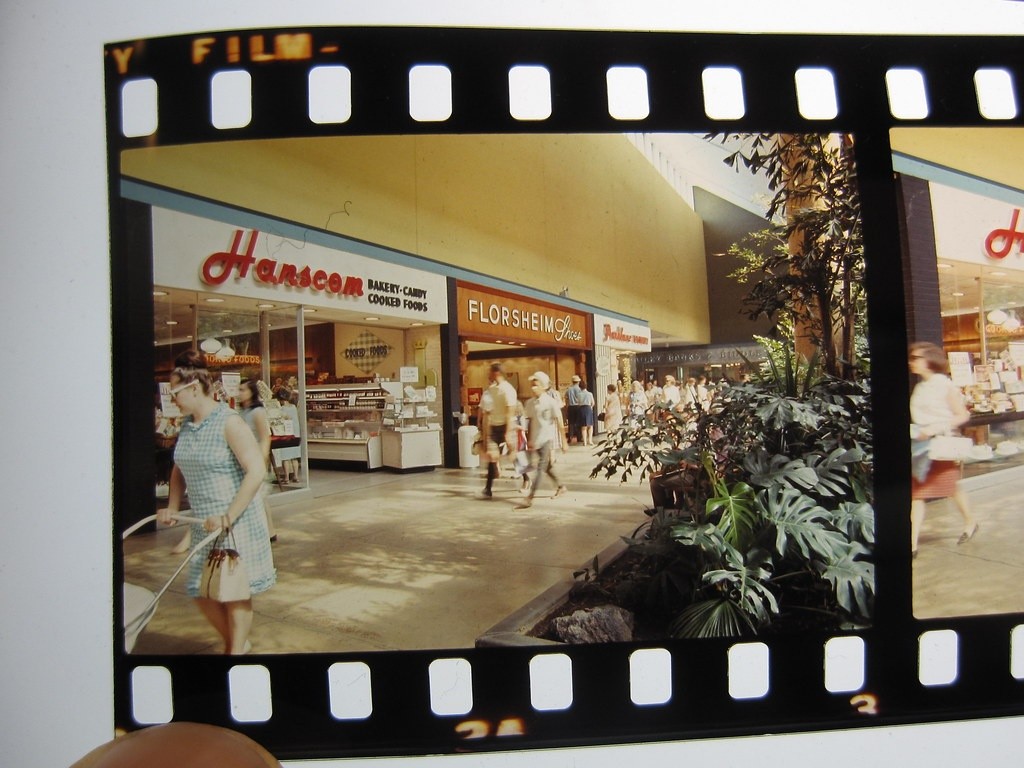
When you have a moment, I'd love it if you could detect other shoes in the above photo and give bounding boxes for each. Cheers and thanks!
[957,522,979,544]
[912,550,918,558]
[270,534,277,542]
[475,491,492,500]
[551,485,567,498]
[513,501,532,509]
[519,478,532,492]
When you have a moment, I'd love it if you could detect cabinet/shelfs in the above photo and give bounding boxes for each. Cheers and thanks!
[305,372,443,470]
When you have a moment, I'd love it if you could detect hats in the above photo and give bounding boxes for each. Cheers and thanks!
[572,375,581,381]
[275,386,291,400]
[528,371,550,389]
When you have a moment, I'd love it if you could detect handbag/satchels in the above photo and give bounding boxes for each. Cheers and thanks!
[598,407,606,421]
[199,514,251,603]
[472,426,538,475]
[694,402,702,410]
[911,439,933,483]
[928,420,970,461]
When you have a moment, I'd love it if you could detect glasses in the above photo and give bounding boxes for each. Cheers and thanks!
[908,355,924,362]
[168,379,199,399]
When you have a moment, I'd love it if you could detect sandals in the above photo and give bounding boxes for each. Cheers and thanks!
[290,478,299,482]
[281,479,289,483]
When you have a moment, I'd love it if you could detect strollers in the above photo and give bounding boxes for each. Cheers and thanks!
[123,512,230,653]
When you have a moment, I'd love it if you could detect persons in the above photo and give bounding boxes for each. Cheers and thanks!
[477,362,532,500]
[577,381,595,447]
[275,389,300,483]
[515,372,568,509]
[564,375,584,443]
[547,386,564,464]
[239,377,277,541]
[909,343,979,557]
[628,375,747,429]
[157,351,275,655]
[603,384,622,435]
